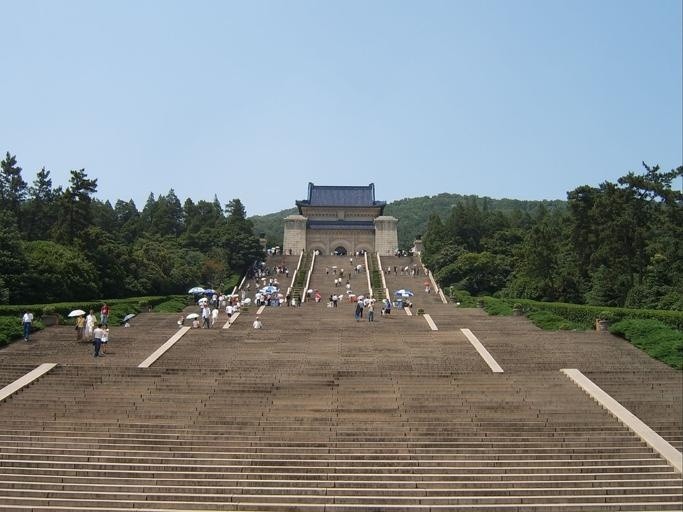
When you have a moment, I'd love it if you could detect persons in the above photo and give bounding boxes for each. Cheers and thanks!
[100,302,110,325]
[21,309,34,342]
[176,244,432,328]
[76,310,109,357]
[124,319,130,328]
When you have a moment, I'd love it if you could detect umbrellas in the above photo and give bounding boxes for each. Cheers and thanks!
[67,309,86,317]
[123,313,136,321]
